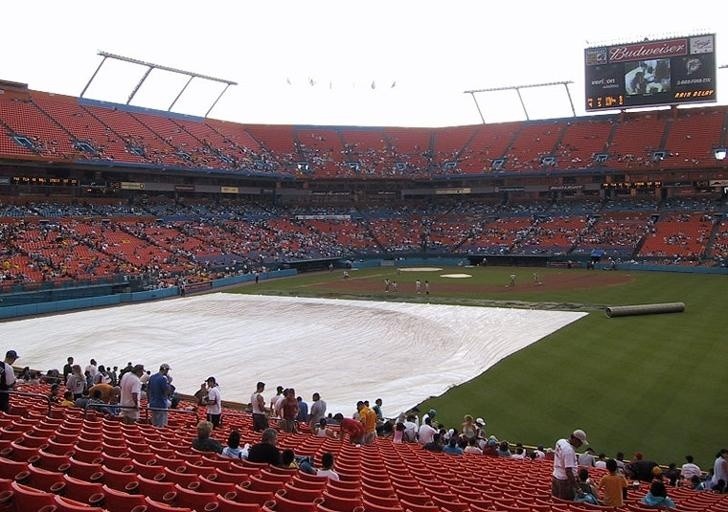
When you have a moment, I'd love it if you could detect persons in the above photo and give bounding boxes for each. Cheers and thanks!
[629,59,672,95]
[1,351,221,430]
[191,420,341,487]
[248,382,552,463]
[2,105,728,297]
[551,429,701,511]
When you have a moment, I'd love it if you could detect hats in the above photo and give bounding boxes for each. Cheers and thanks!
[135,365,147,372]
[332,412,345,420]
[204,377,216,383]
[5,349,21,360]
[160,363,174,371]
[412,406,421,413]
[475,417,487,426]
[427,409,438,416]
[572,429,591,447]
[631,451,644,460]
